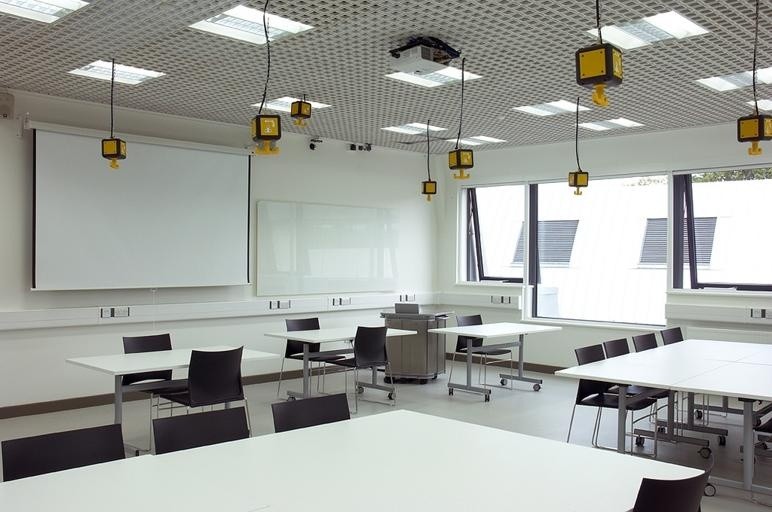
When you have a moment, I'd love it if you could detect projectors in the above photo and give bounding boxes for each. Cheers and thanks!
[389,37,462,76]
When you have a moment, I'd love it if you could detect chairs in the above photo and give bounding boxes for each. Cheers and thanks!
[632,331,710,427]
[1,422,126,482]
[604,337,681,445]
[323,325,397,414]
[156,344,252,448]
[660,326,728,418]
[277,316,349,402]
[150,406,250,457]
[566,343,657,461]
[271,391,350,434]
[120,333,190,451]
[631,453,717,511]
[447,313,513,401]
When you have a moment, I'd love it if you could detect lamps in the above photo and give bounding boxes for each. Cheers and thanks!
[568,96,589,196]
[447,58,474,179]
[575,0,624,107]
[737,0,772,156]
[100,57,126,167]
[290,95,312,128]
[420,119,437,202]
[249,1,283,156]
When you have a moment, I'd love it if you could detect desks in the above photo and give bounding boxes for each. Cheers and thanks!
[261,324,418,403]
[64,344,284,424]
[427,321,563,404]
[1,410,707,512]
[554,337,772,499]
